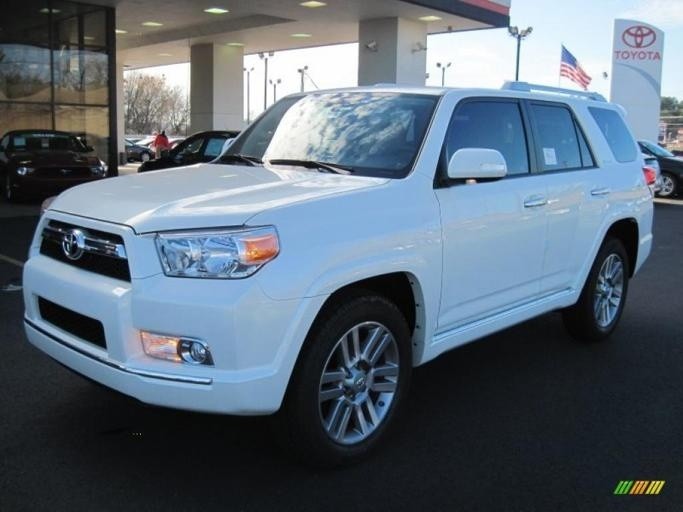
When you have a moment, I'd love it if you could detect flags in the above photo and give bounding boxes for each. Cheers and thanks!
[559,45,593,91]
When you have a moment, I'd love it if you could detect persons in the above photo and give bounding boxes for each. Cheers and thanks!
[154,130,169,159]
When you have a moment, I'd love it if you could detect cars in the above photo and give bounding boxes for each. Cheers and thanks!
[123,127,243,179]
[637,139,683,199]
[0,130,108,202]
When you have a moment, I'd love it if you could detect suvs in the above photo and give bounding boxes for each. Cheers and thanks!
[21,81,659,451]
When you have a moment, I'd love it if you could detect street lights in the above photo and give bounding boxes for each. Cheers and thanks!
[434,61,455,88]
[505,26,534,80]
[240,49,285,125]
[295,65,307,91]
